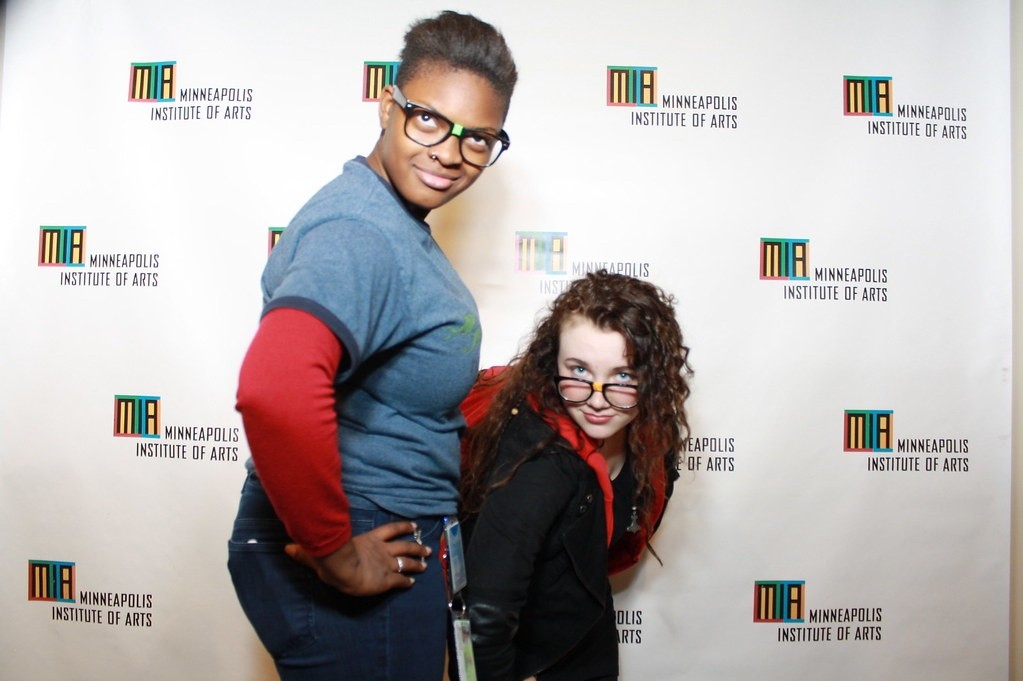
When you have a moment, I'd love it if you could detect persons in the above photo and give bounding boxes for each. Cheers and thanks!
[457,266,693,681]
[226,9,519,681]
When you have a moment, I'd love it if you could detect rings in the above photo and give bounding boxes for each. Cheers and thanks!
[395,557,403,572]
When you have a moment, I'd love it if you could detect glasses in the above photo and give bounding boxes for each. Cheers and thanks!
[552,370,643,411]
[393,84,510,168]
